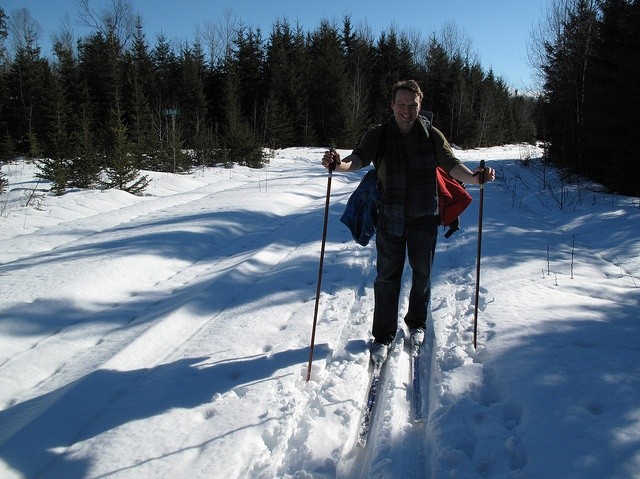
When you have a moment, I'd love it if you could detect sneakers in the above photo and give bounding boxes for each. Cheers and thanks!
[409,327,425,345]
[371,339,391,364]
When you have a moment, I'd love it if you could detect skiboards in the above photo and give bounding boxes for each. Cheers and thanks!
[355,335,423,449]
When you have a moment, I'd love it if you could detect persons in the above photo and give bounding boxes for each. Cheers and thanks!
[321,79,496,365]
